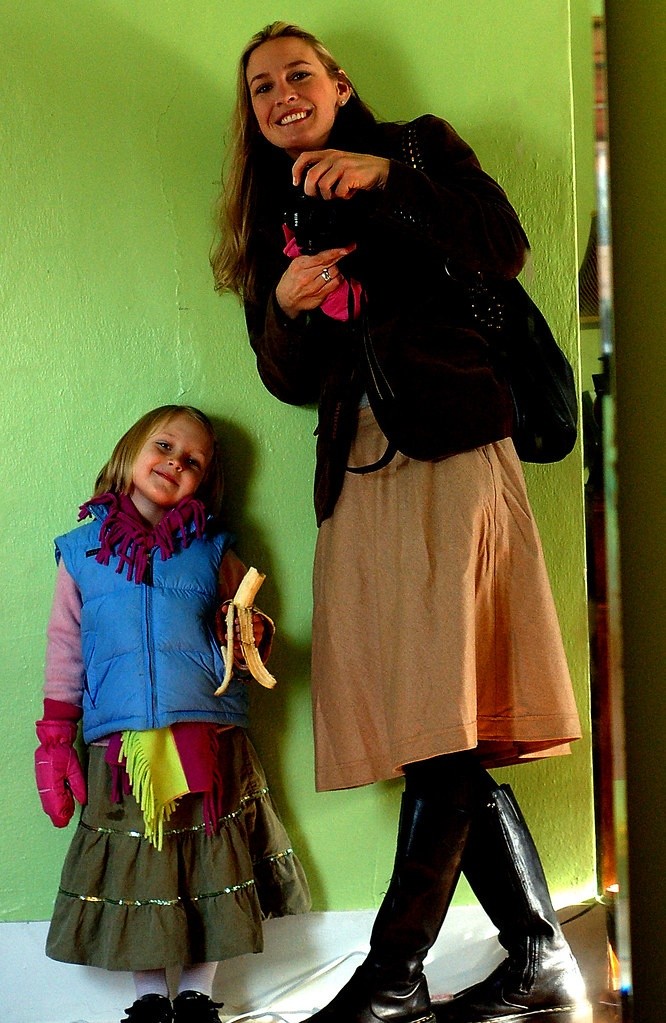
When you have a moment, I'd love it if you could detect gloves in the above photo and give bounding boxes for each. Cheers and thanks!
[35,698,87,827]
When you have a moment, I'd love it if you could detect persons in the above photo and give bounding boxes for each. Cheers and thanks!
[35,405,313,1023]
[211,21,592,1023]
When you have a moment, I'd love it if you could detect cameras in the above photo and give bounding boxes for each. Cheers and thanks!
[285,164,353,255]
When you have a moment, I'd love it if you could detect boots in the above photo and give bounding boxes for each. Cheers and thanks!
[300,791,472,1023]
[433,783,593,1023]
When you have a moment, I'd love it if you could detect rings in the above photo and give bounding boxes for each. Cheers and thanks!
[322,268,331,283]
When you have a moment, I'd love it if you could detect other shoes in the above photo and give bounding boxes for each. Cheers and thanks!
[172,990,224,1023]
[121,993,173,1023]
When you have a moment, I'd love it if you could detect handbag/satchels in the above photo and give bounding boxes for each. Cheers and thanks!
[487,277,578,465]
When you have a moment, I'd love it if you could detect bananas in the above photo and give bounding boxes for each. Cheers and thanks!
[231,566,266,610]
